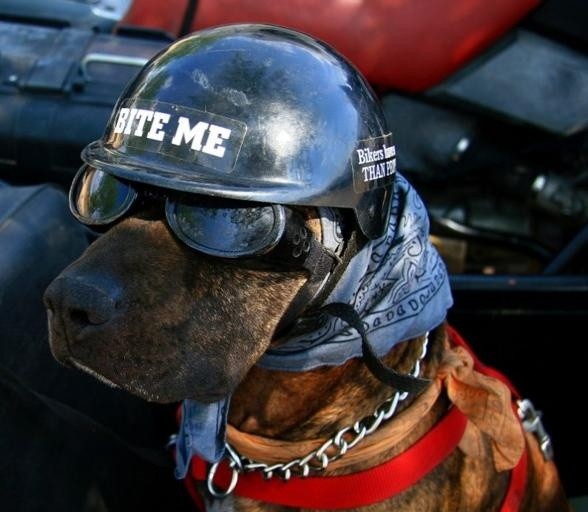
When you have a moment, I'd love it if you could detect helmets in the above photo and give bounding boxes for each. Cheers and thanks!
[80,22,398,243]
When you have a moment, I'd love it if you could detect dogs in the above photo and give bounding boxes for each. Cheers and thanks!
[37,22,572,512]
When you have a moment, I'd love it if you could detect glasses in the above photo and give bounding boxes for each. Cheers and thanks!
[67,154,316,273]
